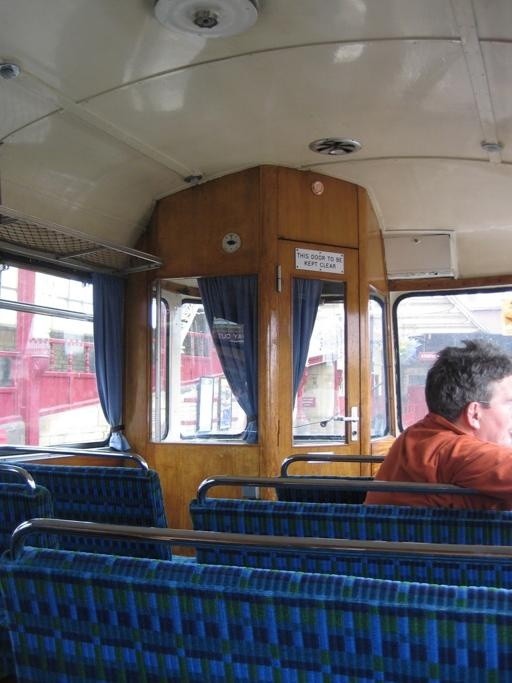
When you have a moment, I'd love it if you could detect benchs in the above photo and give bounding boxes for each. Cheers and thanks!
[0,450,512,681]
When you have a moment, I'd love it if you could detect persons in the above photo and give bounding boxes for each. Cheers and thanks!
[365,340,512,511]
